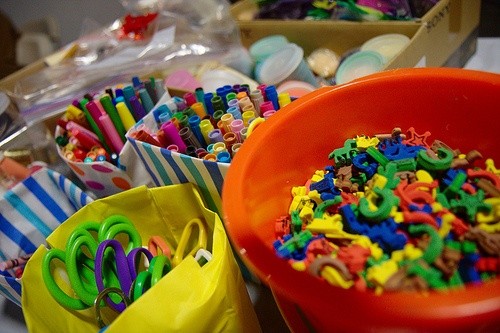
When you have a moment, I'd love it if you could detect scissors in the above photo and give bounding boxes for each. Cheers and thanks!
[40,212,211,330]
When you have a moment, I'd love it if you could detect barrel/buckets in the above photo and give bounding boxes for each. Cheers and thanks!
[223,66,500,333]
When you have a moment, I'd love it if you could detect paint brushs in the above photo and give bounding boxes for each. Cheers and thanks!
[53,76,292,168]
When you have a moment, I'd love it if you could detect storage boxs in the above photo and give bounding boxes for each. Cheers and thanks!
[228,0,480,72]
[0,19,189,124]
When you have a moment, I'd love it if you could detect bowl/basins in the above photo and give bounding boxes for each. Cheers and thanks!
[164,33,411,99]
